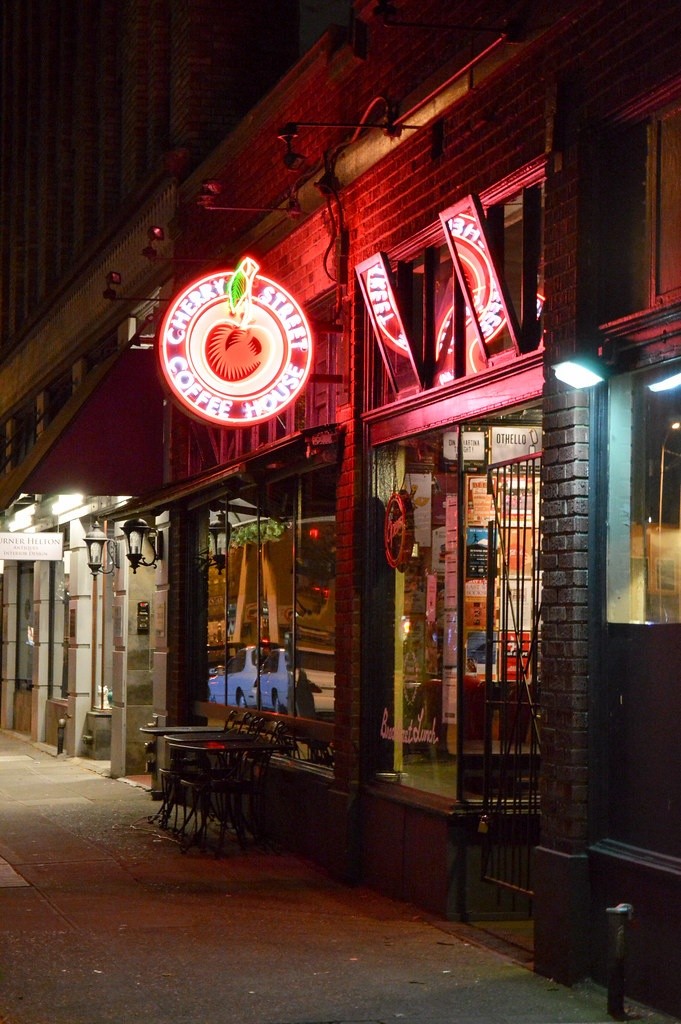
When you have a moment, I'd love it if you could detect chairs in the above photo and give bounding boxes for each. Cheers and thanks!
[149,710,297,858]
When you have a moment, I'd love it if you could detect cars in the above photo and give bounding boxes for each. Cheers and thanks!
[207,634,323,718]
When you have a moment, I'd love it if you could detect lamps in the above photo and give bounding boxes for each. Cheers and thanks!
[372,1,525,42]
[549,352,620,391]
[82,520,122,581]
[276,120,397,136]
[117,509,163,574]
[102,270,169,303]
[191,507,231,576]
[194,178,304,217]
[141,227,237,265]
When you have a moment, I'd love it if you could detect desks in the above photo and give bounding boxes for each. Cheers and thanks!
[140,724,294,850]
